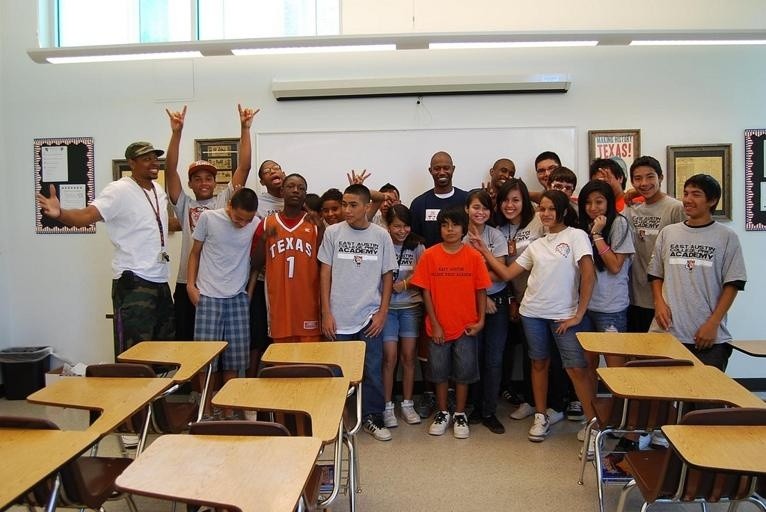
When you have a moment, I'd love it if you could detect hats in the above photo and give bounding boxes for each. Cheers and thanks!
[124,143,165,158]
[187,159,218,176]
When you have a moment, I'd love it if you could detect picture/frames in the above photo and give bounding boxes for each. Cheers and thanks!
[193,137,241,197]
[588,128,641,192]
[34,137,96,235]
[666,142,733,222]
[744,128,766,231]
[111,158,175,235]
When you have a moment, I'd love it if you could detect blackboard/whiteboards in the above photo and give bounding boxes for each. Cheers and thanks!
[253,126,578,208]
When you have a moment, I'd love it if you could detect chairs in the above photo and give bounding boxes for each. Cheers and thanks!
[0,428,102,512]
[576,331,704,486]
[616,423,766,512]
[0,374,175,510]
[85,341,229,434]
[189,377,350,511]
[258,339,367,496]
[593,366,766,512]
[113,433,322,512]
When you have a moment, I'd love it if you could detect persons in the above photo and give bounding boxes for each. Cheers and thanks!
[588,157,645,213]
[489,158,516,188]
[186,188,262,420]
[468,189,603,460]
[461,189,509,434]
[381,203,422,428]
[409,205,493,440]
[317,184,393,441]
[243,159,288,379]
[639,173,748,450]
[576,178,636,441]
[613,156,686,451]
[303,191,321,226]
[251,173,323,343]
[495,178,564,424]
[527,151,579,206]
[320,169,385,225]
[372,181,402,231]
[37,141,177,353]
[544,167,585,422]
[163,104,260,403]
[408,151,469,419]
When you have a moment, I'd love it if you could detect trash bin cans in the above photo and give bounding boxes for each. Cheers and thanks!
[1,345,53,400]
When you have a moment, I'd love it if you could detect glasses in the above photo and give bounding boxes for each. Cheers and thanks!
[262,165,280,175]
[537,166,555,175]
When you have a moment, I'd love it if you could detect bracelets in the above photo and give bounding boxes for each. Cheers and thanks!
[591,232,602,236]
[403,279,407,291]
[598,246,610,255]
[593,238,604,242]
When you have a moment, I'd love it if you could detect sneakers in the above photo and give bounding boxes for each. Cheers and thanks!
[401,400,421,424]
[447,388,456,409]
[417,392,436,418]
[428,411,452,435]
[579,429,606,461]
[362,415,392,440]
[453,412,469,439]
[468,409,482,424]
[501,388,523,405]
[568,401,585,421]
[383,402,398,427]
[577,426,587,442]
[527,413,552,441]
[482,415,505,434]
[546,409,564,424]
[510,403,537,419]
